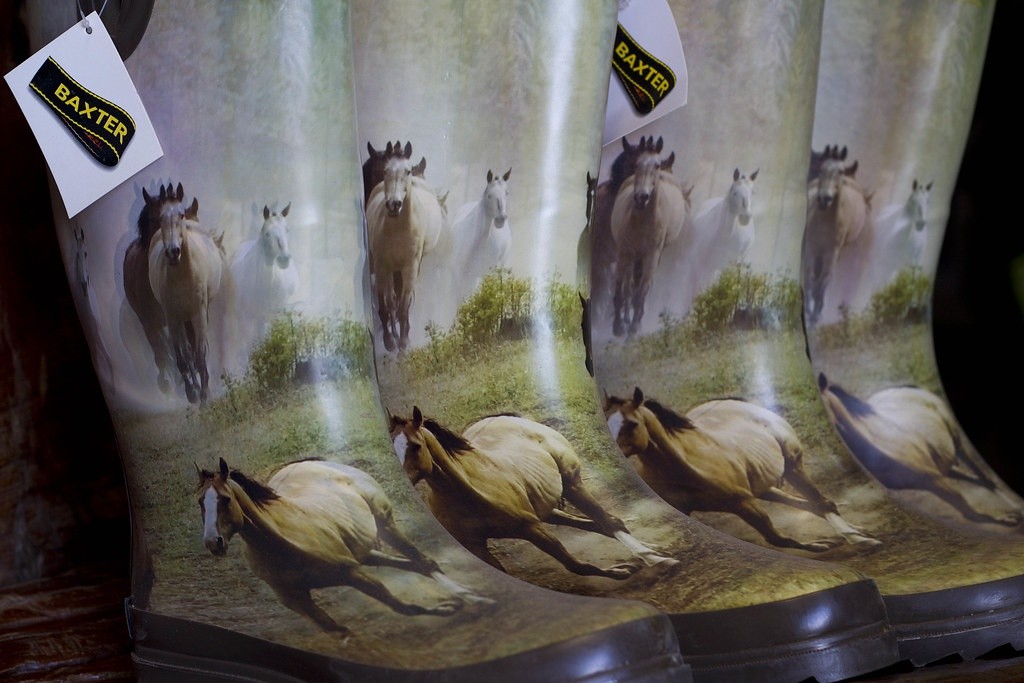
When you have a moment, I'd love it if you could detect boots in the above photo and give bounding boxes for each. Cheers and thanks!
[583,0,1024,665]
[28,0,694,683]
[347,0,900,683]
[811,0,1024,537]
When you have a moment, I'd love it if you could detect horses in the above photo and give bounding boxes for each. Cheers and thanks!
[123,139,1015,646]
